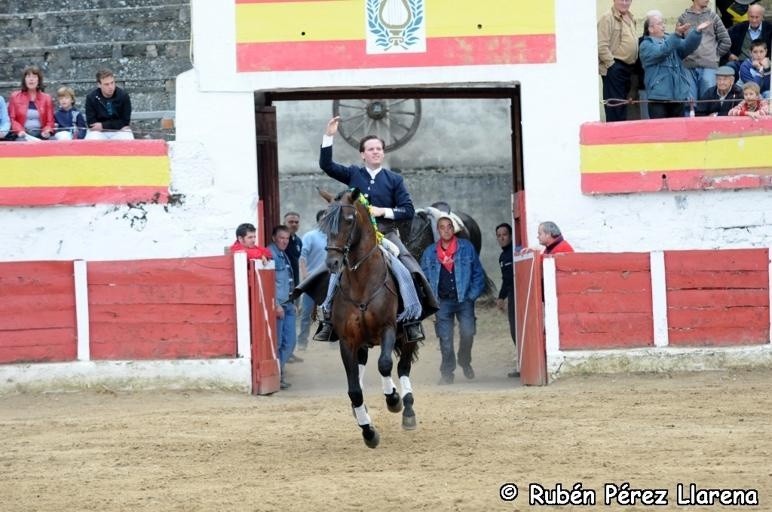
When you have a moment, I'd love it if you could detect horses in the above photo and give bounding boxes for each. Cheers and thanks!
[312,186,426,449]
[405,208,498,352]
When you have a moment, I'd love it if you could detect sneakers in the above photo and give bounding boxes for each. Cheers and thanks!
[288,345,306,364]
[313,323,330,341]
[507,371,520,377]
[437,375,454,386]
[464,363,475,379]
[409,324,425,340]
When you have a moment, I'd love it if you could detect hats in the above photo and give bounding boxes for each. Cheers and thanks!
[716,66,735,76]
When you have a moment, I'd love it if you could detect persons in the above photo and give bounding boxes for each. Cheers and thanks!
[495,223,521,378]
[282,212,304,363]
[597,0,772,121]
[83,69,134,140]
[417,217,488,385]
[230,223,272,267]
[0,96,11,139]
[7,66,56,142]
[266,225,298,390]
[519,220,575,358]
[285,114,440,342]
[53,85,86,140]
[296,208,327,350]
